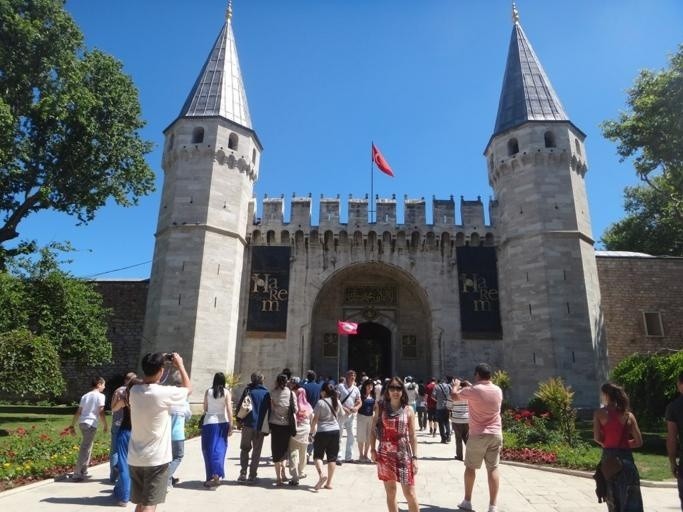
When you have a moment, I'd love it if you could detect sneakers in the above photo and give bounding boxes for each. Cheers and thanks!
[72,478,82,482]
[457,500,472,511]
[172,476,179,486]
[204,473,307,488]
[116,501,127,507]
[418,427,451,444]
[336,456,371,465]
[110,478,117,483]
[488,505,498,512]
[81,472,87,476]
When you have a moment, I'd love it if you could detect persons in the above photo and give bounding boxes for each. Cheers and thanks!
[167,369,192,488]
[126,352,193,512]
[111,377,143,507]
[665,370,683,512]
[201,372,233,487]
[593,384,644,512]
[110,372,138,485]
[268,368,469,512]
[235,372,271,484]
[70,377,108,482]
[451,363,503,512]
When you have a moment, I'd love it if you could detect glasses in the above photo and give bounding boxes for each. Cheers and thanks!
[389,385,401,392]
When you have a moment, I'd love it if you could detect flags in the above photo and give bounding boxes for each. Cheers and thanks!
[372,142,394,177]
[337,319,359,335]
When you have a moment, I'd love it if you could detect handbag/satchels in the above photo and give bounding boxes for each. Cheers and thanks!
[198,415,205,429]
[236,395,252,419]
[446,401,452,409]
[288,392,297,437]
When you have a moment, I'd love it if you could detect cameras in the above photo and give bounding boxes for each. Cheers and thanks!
[162,354,176,361]
[457,380,469,388]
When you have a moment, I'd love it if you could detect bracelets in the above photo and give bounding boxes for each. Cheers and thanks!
[236,420,240,422]
